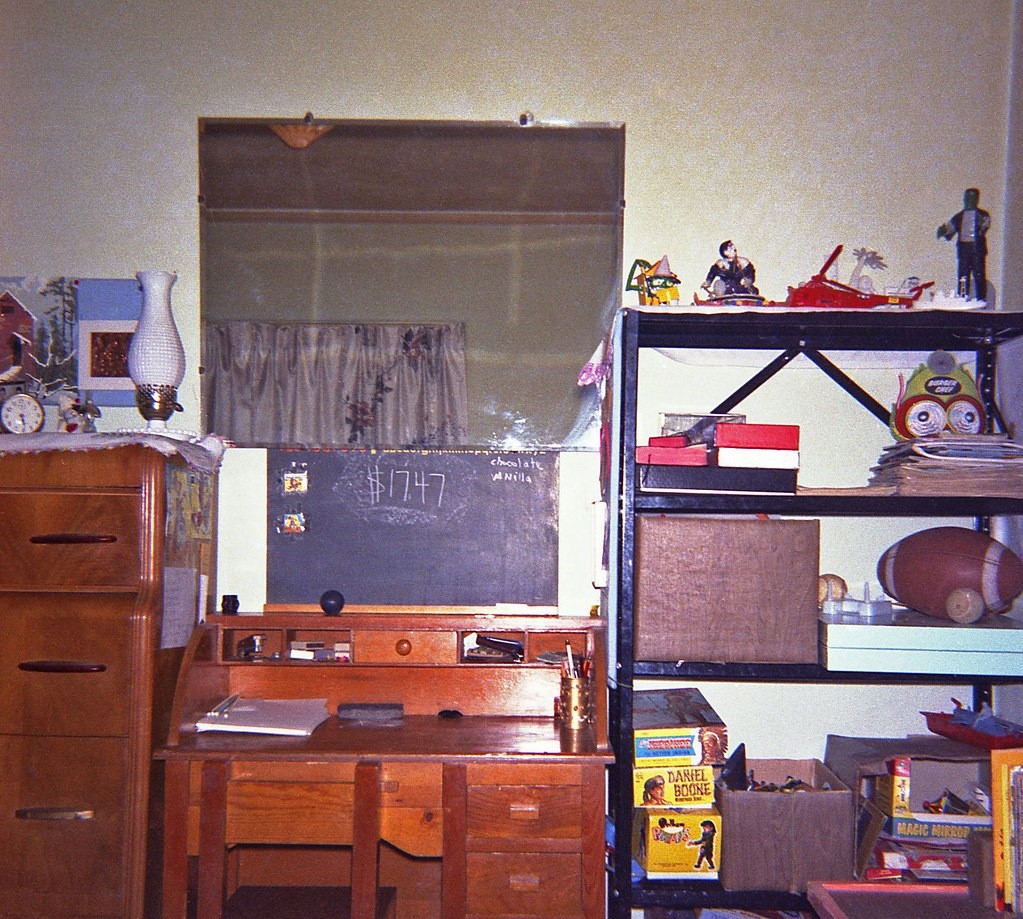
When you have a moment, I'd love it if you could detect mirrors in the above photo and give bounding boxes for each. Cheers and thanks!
[196,115,624,450]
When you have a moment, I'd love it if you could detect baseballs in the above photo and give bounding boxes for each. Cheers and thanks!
[945,588,983,624]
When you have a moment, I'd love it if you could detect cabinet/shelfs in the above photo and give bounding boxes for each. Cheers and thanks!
[606,305,1023,918]
[0,433,600,918]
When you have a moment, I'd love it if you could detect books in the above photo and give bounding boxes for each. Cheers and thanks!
[870,434,1022,467]
[196,696,329,737]
[991,748,1023,919]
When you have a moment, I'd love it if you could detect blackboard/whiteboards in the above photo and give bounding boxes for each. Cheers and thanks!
[264,443,554,630]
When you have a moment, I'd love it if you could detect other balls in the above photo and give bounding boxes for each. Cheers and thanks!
[818,574,846,609]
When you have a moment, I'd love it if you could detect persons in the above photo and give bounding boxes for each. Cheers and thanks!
[938,188,990,309]
[702,240,758,297]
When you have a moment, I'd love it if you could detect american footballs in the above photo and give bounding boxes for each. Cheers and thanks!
[875,525,1023,620]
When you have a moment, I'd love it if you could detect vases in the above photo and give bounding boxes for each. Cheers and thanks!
[127,269,185,431]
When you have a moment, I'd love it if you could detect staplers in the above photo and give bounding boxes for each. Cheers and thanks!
[235,633,268,662]
[465,635,524,663]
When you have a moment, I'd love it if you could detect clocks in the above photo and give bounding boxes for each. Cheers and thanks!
[0,392,45,434]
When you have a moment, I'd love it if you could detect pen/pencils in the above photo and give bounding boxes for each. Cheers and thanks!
[206,692,239,719]
[559,639,593,678]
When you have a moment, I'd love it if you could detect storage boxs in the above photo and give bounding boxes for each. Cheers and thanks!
[819,607,1023,677]
[714,422,801,470]
[609,688,994,894]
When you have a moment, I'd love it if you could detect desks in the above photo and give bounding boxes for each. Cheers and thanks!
[150,699,616,919]
